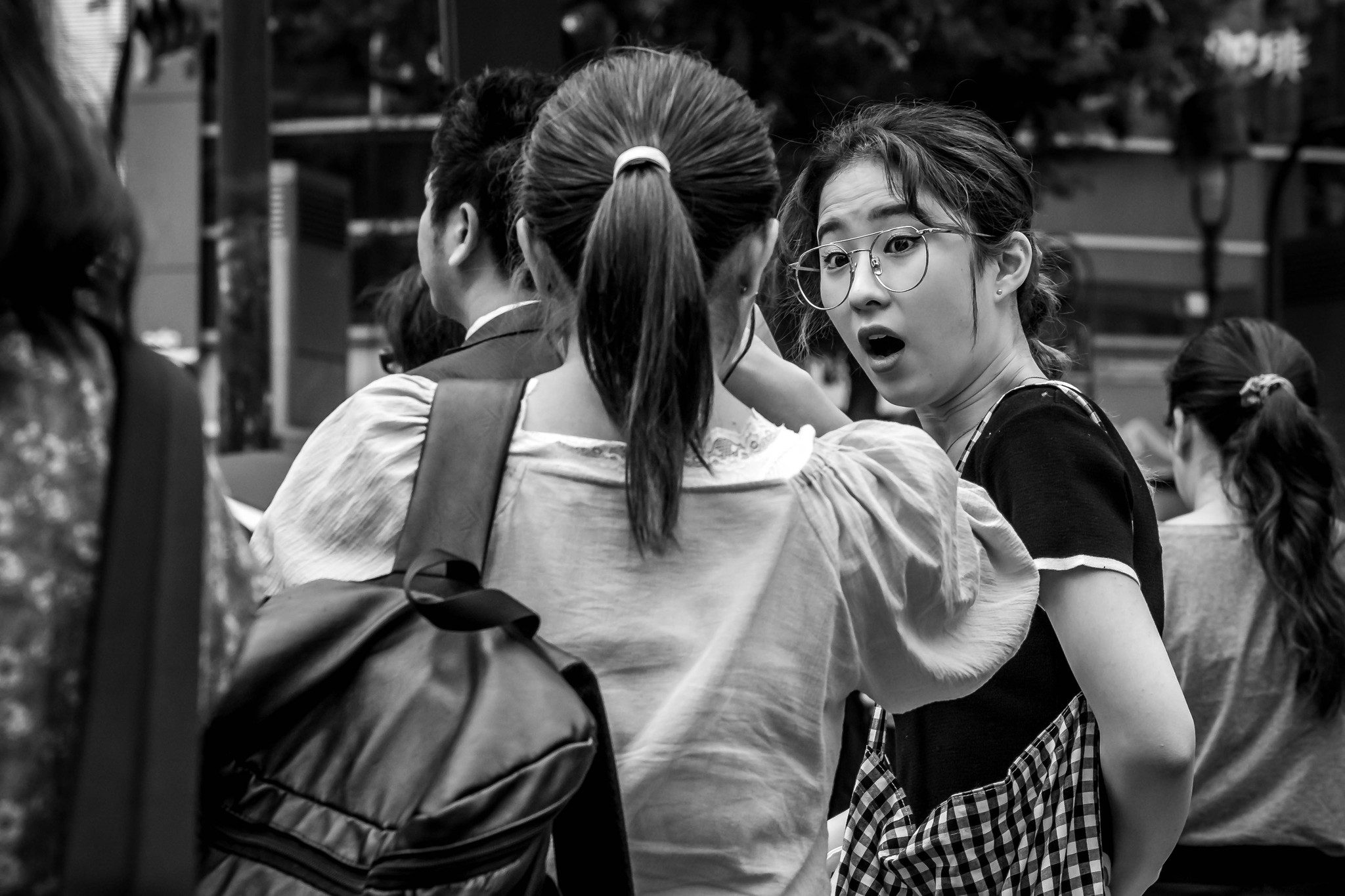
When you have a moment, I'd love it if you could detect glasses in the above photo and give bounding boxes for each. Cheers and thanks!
[788,226,1001,311]
[379,353,404,374]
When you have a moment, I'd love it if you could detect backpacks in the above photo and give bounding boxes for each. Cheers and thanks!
[197,382,635,896]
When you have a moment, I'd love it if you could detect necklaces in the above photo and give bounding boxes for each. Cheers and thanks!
[946,377,1050,452]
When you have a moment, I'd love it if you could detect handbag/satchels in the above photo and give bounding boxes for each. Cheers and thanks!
[831,381,1110,896]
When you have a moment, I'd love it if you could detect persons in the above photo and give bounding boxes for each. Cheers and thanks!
[0,0,256,896]
[251,47,1041,896]
[375,68,1345,896]
